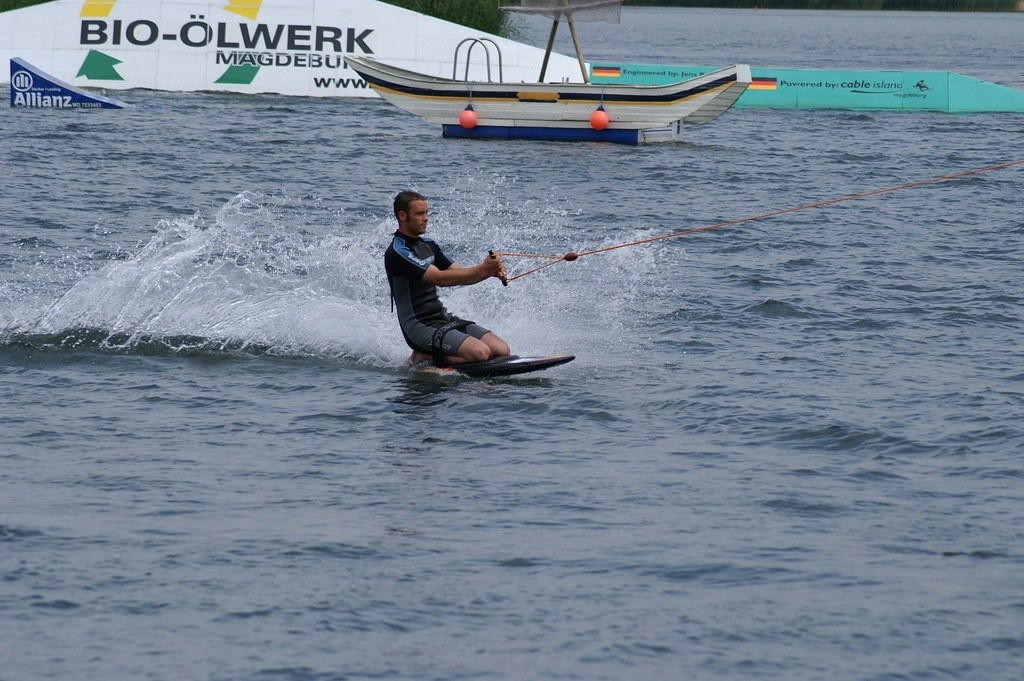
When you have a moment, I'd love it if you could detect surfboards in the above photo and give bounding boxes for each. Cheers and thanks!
[440,354,576,378]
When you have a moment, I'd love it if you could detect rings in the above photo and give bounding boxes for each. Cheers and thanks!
[504,272,506,275]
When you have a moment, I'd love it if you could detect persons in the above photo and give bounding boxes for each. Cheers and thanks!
[384,191,509,364]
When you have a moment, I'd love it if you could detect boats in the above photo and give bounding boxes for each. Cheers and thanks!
[342,1,753,146]
